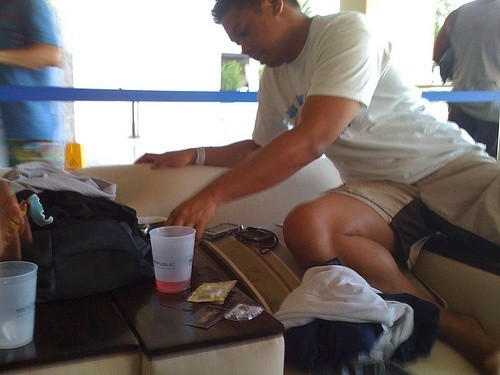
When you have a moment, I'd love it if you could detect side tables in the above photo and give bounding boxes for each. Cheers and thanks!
[0,237,286,375]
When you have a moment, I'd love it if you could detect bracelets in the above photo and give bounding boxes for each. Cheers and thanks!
[195,147,205,165]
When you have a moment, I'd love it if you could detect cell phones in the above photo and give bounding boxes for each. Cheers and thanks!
[204,223,240,239]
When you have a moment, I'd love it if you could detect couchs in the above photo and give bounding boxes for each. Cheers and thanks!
[71,157,500,375]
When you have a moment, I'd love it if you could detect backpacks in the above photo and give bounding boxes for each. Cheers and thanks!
[16,189,154,302]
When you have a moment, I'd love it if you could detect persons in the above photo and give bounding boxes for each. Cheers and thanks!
[0,0,66,169]
[432,0,500,159]
[134,0,500,375]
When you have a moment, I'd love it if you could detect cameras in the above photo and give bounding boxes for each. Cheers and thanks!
[236,227,275,248]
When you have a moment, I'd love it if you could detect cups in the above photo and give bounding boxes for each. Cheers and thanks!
[0,261,38,349]
[149,226,196,293]
